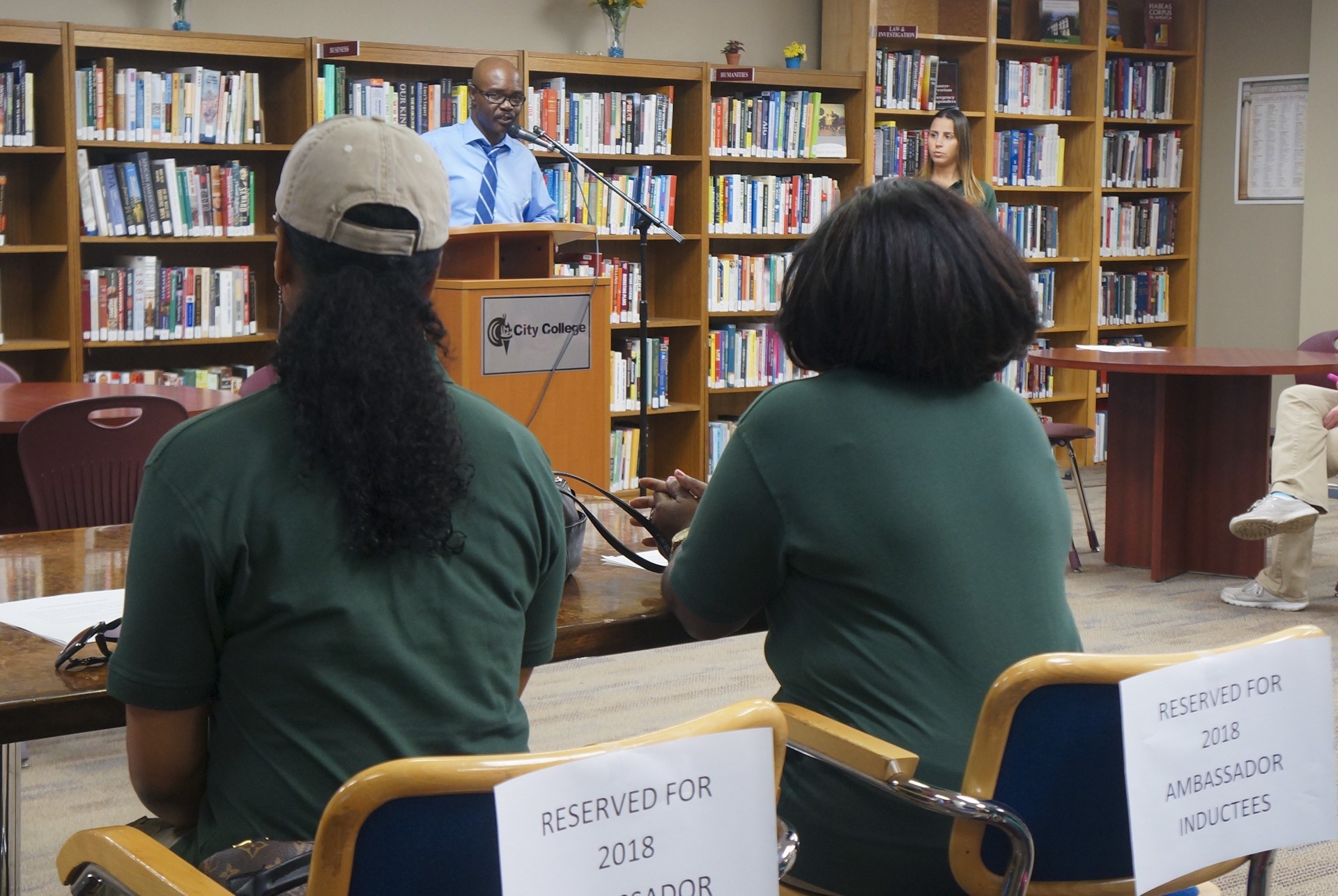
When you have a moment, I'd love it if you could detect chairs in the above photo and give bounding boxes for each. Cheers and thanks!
[57,650,1276,895]
[0,360,188,532]
[1041,423,1100,576]
[1295,330,1338,389]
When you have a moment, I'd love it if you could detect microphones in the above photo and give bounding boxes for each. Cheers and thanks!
[507,123,562,154]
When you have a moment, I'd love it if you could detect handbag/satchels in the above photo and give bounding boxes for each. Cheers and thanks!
[551,470,672,585]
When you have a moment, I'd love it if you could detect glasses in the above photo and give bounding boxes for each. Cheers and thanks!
[470,84,526,106]
[55,616,123,672]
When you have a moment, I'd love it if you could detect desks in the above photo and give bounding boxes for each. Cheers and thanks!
[1025,346,1338,582]
[0,383,242,437]
[0,492,691,896]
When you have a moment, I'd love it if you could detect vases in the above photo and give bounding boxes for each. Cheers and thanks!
[785,56,801,69]
[170,0,190,31]
[600,6,630,58]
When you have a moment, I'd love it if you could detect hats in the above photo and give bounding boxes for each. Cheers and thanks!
[275,114,452,257]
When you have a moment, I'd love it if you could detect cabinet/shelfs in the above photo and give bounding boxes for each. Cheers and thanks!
[0,0,1209,493]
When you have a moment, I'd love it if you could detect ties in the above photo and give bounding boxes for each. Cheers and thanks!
[473,141,511,225]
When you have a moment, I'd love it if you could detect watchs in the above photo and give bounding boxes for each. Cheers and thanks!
[669,527,690,559]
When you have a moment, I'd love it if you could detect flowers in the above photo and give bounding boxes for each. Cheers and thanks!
[780,41,808,61]
[589,0,648,39]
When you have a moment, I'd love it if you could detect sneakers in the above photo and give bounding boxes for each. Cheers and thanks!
[1229,494,1320,541]
[1220,579,1310,612]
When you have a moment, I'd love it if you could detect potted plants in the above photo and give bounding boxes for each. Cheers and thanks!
[719,40,746,66]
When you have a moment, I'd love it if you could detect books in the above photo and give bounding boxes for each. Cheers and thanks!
[610,333,669,414]
[873,49,960,180]
[0,174,8,244]
[77,145,255,239]
[80,253,258,341]
[74,53,269,143]
[527,77,674,156]
[554,250,642,325]
[0,60,38,147]
[84,363,255,400]
[610,428,642,493]
[538,162,676,236]
[707,0,1183,484]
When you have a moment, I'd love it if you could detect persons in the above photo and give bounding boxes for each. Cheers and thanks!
[628,175,1084,896]
[419,54,558,227]
[103,115,567,859]
[1219,371,1338,612]
[921,105,1000,225]
